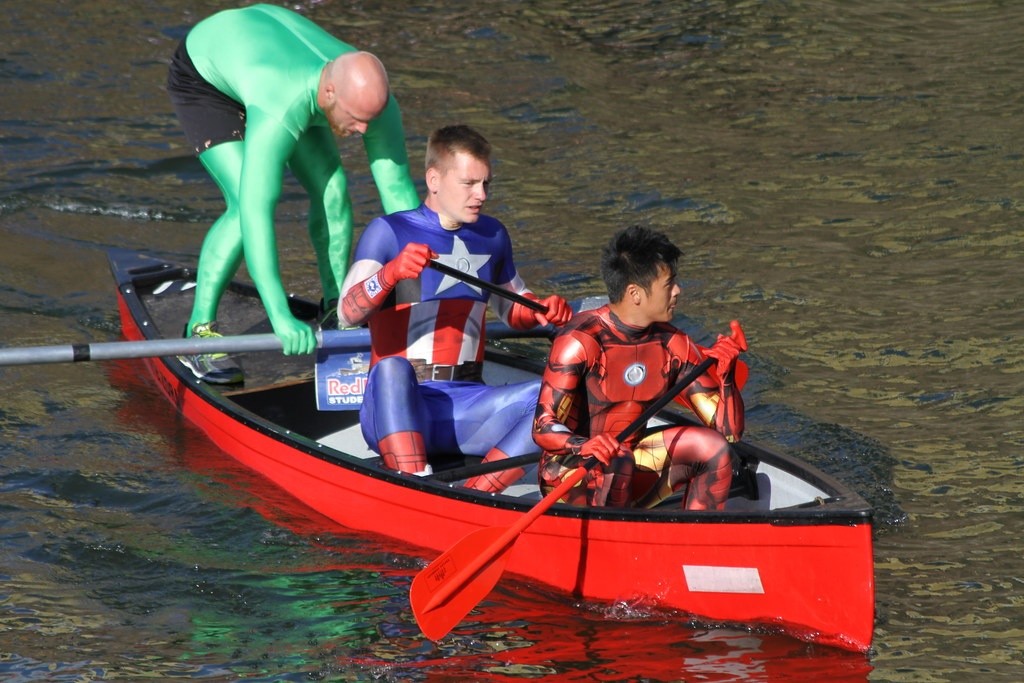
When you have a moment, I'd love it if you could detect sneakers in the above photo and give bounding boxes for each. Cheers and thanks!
[175,324,243,385]
[317,298,363,331]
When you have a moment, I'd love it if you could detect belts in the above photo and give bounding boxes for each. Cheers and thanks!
[412,362,485,386]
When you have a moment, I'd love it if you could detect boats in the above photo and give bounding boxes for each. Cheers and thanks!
[105,248,875,655]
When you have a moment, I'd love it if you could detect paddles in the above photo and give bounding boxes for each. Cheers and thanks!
[426,257,572,328]
[409,317,749,645]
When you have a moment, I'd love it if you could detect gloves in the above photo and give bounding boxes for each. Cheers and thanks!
[580,432,626,467]
[390,243,440,280]
[703,334,740,386]
[273,314,317,355]
[533,294,574,327]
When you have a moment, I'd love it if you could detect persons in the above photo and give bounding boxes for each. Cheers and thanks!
[166,2,420,384]
[336,124,573,494]
[532,226,745,511]
